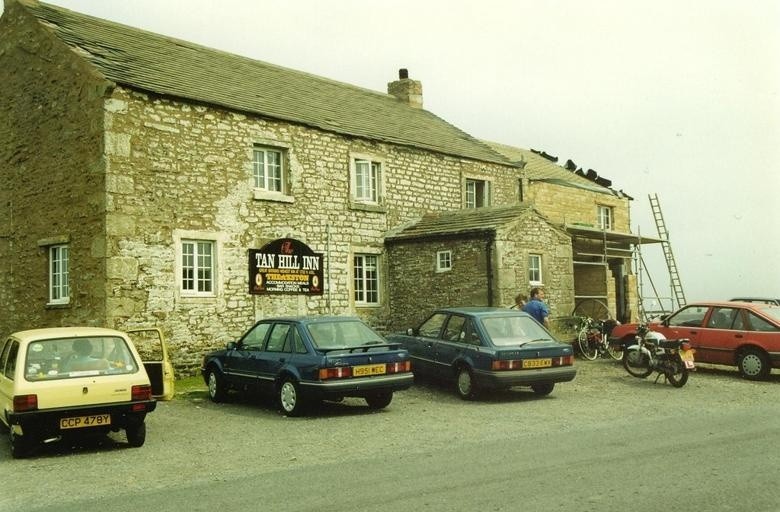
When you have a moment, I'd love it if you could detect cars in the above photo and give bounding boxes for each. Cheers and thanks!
[679,296,780,333]
[0,325,178,461]
[607,301,780,383]
[382,305,579,403]
[198,312,416,419]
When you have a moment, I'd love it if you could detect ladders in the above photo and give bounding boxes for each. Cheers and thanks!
[648,193,687,308]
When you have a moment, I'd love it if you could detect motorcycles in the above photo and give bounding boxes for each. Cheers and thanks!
[620,314,697,388]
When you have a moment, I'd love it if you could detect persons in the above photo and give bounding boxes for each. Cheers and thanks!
[522,287,551,330]
[60,338,95,373]
[510,294,529,310]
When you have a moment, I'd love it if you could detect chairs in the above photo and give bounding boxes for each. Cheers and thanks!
[278,332,346,348]
[30,358,112,375]
[713,308,740,330]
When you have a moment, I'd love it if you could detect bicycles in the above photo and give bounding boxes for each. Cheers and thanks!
[570,313,625,362]
